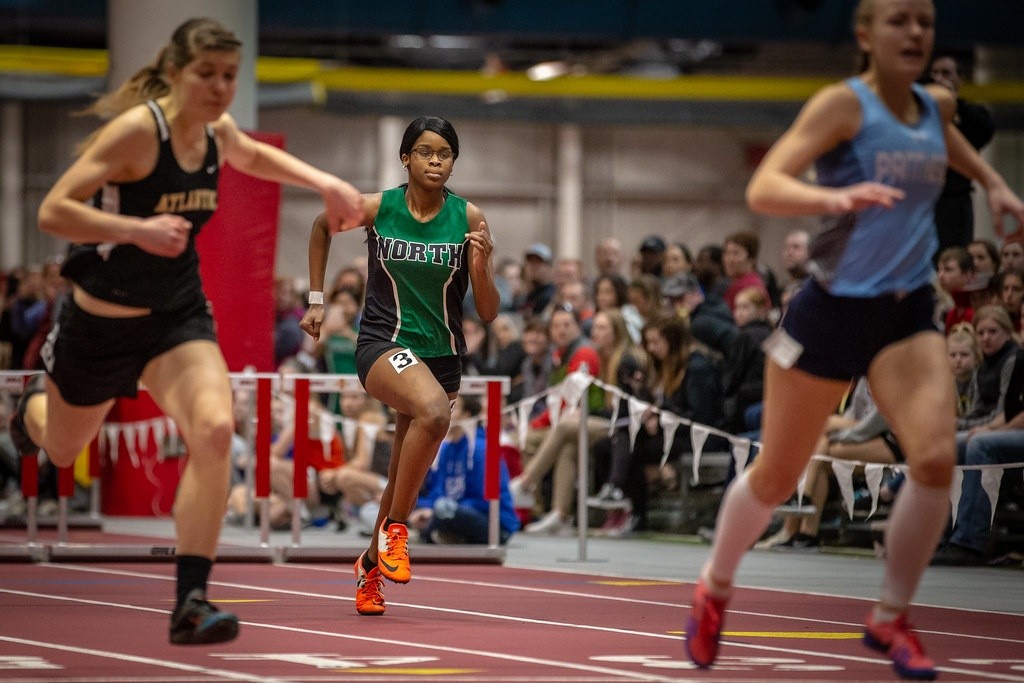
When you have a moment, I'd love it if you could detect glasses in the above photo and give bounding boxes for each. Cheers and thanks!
[408,145,455,160]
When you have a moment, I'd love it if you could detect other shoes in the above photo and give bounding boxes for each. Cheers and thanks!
[508,477,535,508]
[529,511,570,531]
[595,508,638,536]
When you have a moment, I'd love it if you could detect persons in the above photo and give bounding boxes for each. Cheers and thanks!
[299,115,500,613]
[8,17,366,644]
[1,257,89,514]
[930,57,995,266]
[686,0,1024,683]
[233,263,519,546]
[460,230,1023,562]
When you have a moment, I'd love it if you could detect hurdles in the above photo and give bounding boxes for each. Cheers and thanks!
[0,369,512,562]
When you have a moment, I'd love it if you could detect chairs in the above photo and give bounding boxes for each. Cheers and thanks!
[644,393,786,531]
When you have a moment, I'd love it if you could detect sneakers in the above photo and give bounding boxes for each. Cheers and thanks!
[10,372,48,456]
[863,610,934,678]
[685,574,728,667]
[353,549,386,615]
[377,517,411,584]
[170,589,238,645]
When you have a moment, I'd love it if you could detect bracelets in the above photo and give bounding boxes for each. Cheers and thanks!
[309,292,324,305]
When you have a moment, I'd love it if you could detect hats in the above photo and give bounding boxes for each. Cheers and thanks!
[525,242,552,262]
